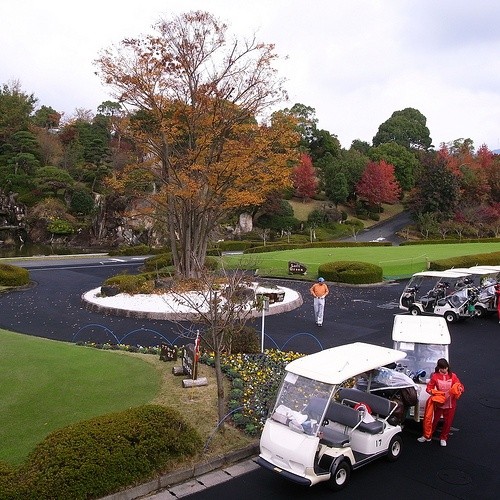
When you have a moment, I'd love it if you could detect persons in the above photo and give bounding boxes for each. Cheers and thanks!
[496,282,500,324]
[417,358,462,446]
[309,277,329,326]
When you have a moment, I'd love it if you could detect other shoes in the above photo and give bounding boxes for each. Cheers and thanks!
[417,436,431,442]
[441,440,446,446]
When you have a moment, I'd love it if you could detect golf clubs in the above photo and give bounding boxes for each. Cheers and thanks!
[393,362,414,378]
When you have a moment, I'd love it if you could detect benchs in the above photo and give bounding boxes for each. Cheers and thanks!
[338,388,398,434]
[419,289,442,303]
[437,294,454,306]
[309,397,363,448]
